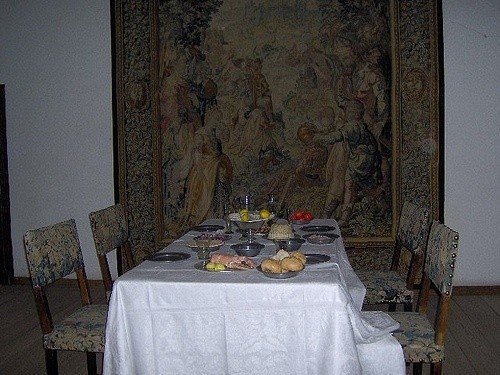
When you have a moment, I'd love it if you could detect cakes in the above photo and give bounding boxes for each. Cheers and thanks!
[267,223,294,239]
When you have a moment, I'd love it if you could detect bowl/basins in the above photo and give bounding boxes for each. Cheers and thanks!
[186,243,225,252]
[303,235,339,244]
[278,238,304,250]
[255,264,305,279]
[293,218,312,225]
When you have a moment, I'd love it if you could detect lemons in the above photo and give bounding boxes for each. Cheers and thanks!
[240,209,249,222]
[260,209,270,218]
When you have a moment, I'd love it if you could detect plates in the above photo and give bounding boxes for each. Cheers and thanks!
[192,224,225,230]
[303,254,331,266]
[146,252,190,263]
[193,260,244,272]
[300,226,336,231]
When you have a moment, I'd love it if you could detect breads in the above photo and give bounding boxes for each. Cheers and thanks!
[261,250,306,274]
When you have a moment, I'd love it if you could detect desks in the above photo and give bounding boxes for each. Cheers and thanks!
[103,220,406,375]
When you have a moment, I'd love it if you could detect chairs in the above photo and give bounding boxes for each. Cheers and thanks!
[355,201,431,311]
[88,204,136,299]
[385,219,459,375]
[23,220,108,375]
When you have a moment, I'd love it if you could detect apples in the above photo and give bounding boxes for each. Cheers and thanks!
[288,211,312,221]
